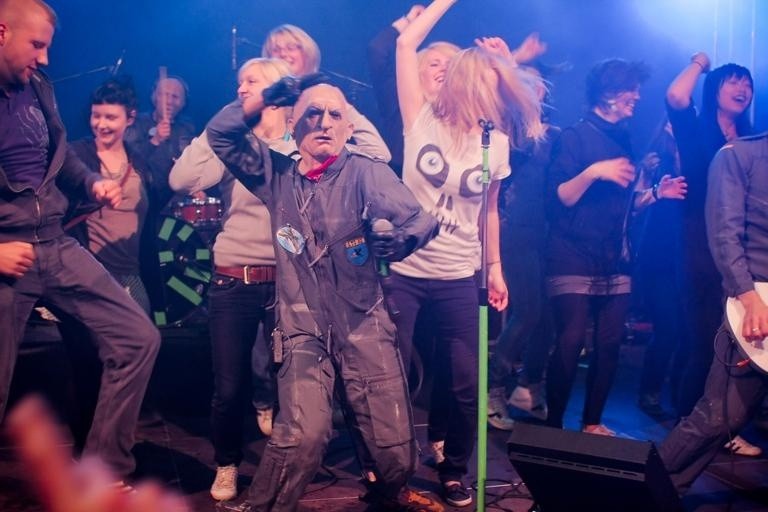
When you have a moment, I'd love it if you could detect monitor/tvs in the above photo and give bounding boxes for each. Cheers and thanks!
[507,422,678,512]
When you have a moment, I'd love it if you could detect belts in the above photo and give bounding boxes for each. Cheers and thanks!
[215,264,277,286]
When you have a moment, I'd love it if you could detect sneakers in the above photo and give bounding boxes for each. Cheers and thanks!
[582,424,617,437]
[440,480,473,507]
[638,393,666,417]
[256,406,273,436]
[392,485,447,512]
[209,463,241,502]
[486,386,517,431]
[507,383,549,421]
[724,434,762,457]
[427,438,446,464]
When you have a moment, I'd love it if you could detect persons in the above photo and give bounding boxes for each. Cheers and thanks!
[0,1,767,512]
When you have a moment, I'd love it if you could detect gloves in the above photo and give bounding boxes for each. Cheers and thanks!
[261,71,331,107]
[371,233,417,262]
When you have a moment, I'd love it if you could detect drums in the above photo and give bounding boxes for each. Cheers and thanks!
[173,196,222,228]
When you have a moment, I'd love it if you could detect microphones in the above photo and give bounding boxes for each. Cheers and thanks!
[231,24,238,71]
[371,219,395,277]
[111,47,127,76]
